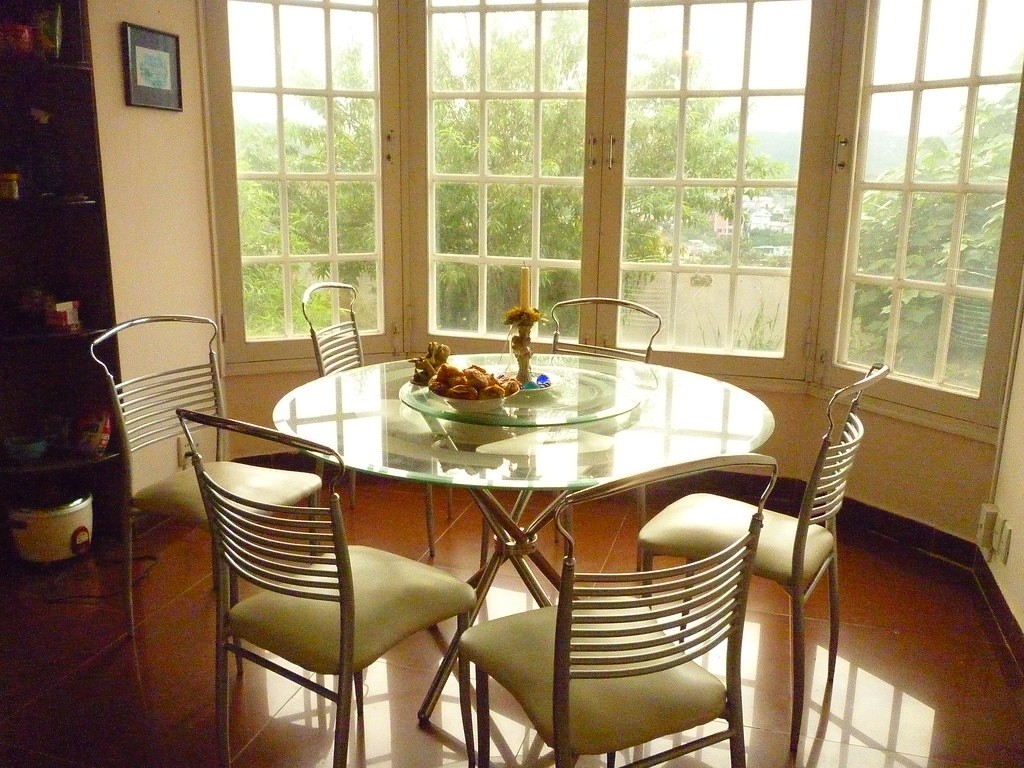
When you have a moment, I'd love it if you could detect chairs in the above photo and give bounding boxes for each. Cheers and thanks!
[300,280,452,562]
[90,315,321,676]
[176,408,476,768]
[458,454,779,768]
[637,362,889,751]
[478,295,663,567]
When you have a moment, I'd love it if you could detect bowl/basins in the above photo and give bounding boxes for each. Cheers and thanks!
[439,385,518,414]
[4,436,48,463]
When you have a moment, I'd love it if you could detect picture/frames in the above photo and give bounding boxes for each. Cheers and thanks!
[122,21,184,111]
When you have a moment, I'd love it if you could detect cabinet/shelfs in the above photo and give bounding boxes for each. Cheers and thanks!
[0,0,122,476]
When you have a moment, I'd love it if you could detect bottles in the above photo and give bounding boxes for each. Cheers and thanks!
[0,172,19,200]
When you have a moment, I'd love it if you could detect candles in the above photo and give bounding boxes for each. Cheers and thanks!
[520,259,529,311]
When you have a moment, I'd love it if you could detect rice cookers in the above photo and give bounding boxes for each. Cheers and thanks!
[7,489,94,562]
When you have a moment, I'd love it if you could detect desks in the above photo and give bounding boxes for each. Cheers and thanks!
[273,354,775,720]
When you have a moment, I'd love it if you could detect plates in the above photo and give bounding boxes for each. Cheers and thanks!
[504,372,560,394]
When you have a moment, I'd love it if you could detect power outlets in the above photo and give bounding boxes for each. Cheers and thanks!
[177,430,200,467]
